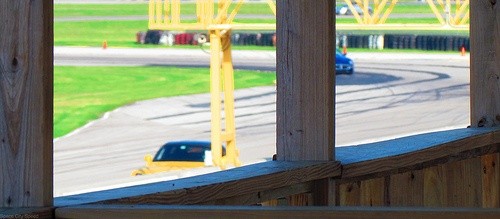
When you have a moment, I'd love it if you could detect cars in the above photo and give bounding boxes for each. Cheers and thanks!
[334,49,353,75]
[136,140,228,179]
